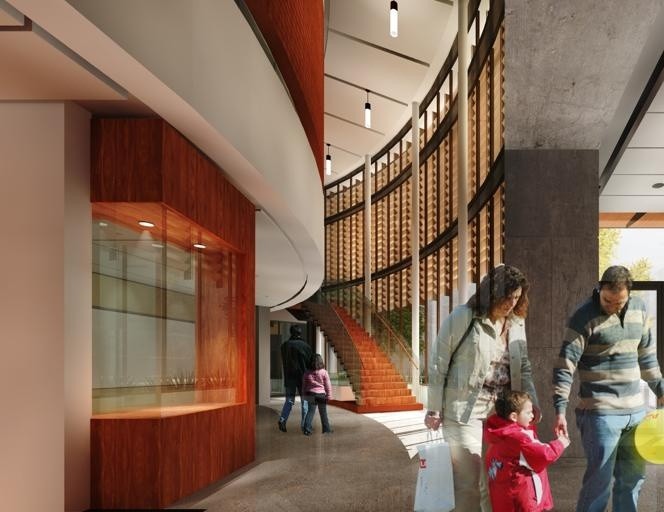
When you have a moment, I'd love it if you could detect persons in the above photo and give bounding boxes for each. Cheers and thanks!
[482,391,570,511]
[303,353,334,435]
[423,264,541,511]
[551,266,663,511]
[276,324,314,433]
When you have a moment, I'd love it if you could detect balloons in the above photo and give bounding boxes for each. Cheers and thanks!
[633,408,663,464]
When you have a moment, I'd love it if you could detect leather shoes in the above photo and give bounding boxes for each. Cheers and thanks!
[303,426,313,435]
[278,417,287,431]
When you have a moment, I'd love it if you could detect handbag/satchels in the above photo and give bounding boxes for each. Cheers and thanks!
[412,427,457,512]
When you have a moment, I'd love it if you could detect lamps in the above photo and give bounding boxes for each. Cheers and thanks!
[324,144,331,175]
[363,88,372,128]
[388,1,401,39]
